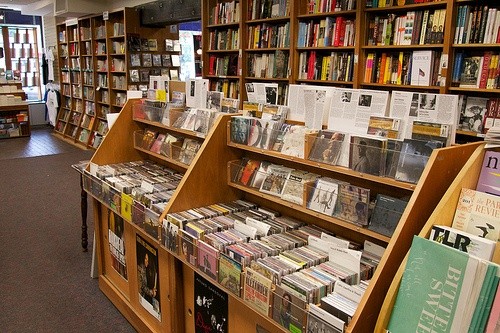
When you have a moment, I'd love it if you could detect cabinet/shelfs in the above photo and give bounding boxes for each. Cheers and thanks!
[0,0,500,333]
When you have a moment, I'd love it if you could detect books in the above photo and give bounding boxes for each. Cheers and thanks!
[0,0,500,332]
